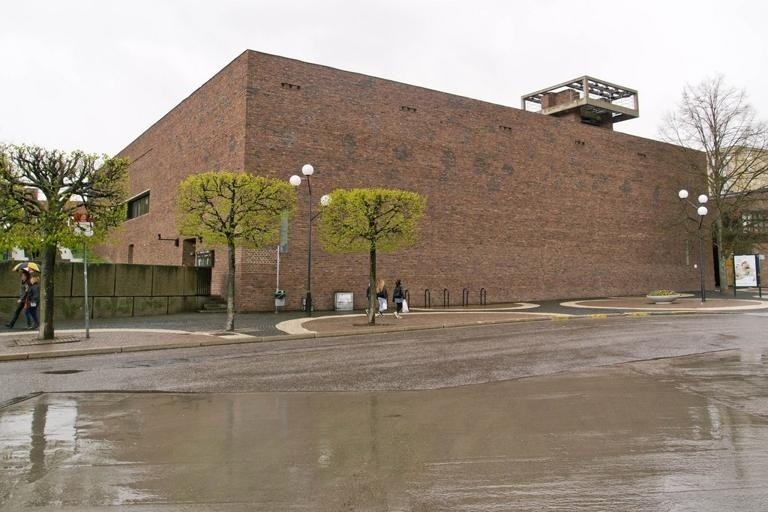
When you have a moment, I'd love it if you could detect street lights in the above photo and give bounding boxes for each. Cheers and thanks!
[72,227,93,338]
[679,189,708,302]
[290,164,332,316]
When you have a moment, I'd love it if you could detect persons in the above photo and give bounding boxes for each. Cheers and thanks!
[364,282,372,315]
[392,279,406,319]
[375,279,389,316]
[27,276,39,331]
[4,271,32,328]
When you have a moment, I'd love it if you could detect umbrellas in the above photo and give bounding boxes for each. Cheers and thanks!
[12,261,40,273]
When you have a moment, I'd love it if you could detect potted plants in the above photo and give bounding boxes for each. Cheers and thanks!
[646,287,681,305]
